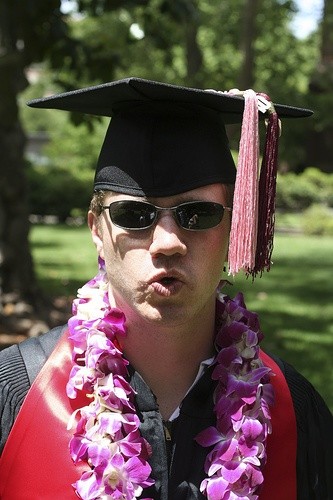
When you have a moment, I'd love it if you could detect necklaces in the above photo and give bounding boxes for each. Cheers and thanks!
[68,255,274,500]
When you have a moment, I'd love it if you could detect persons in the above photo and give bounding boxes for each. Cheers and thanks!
[0,77,333,500]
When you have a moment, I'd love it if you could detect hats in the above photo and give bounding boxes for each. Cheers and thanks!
[26,77,315,284]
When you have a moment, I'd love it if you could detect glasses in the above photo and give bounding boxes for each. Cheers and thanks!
[98,199,233,231]
